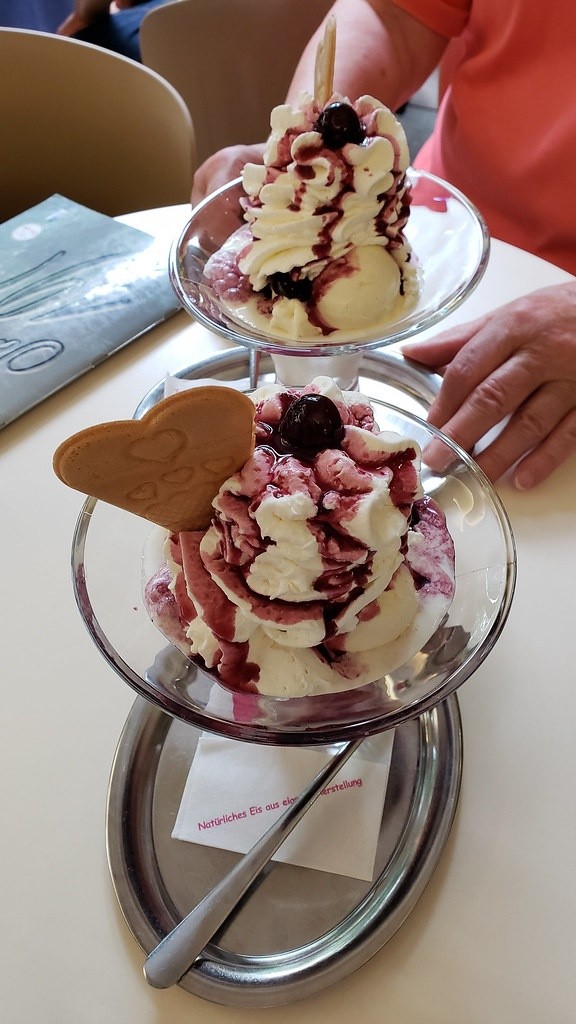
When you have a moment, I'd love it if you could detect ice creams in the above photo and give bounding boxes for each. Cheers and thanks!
[202,12,422,341]
[52,374,455,699]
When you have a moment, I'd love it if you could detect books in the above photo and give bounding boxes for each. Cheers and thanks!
[0,193,184,430]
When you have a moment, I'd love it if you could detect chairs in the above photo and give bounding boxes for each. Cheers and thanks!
[0,26,197,224]
[139,0,336,171]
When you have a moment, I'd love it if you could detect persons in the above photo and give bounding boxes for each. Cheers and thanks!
[0,0,179,63]
[190,0,576,491]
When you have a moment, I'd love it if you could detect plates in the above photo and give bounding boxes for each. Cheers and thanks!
[134,338,472,501]
[166,169,489,357]
[70,397,516,748]
[106,636,460,1009]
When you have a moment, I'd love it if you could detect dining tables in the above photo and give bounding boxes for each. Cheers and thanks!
[0,202,576,1024]
